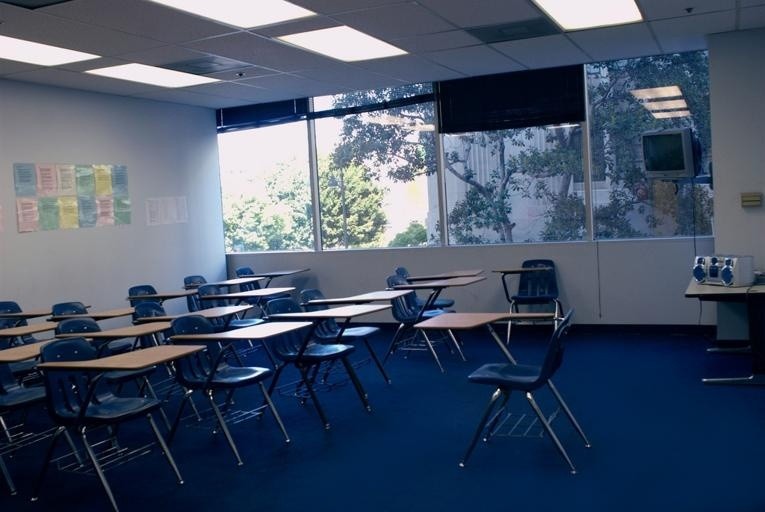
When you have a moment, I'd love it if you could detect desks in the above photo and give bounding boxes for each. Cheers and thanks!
[682,271,765,386]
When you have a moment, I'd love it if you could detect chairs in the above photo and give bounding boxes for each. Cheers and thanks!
[0,259,394,510]
[385,259,592,475]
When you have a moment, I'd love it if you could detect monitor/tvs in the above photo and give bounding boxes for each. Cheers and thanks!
[641,128,701,179]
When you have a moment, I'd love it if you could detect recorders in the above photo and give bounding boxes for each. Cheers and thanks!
[692,255,754,287]
